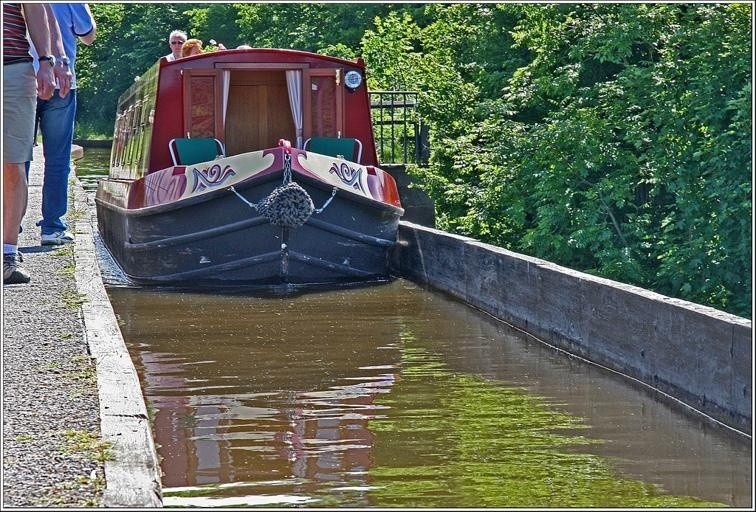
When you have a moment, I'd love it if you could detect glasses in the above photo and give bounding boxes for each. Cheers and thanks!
[171,41,184,44]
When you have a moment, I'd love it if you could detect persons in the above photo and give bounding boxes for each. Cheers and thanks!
[16,4,73,264]
[162,30,187,60]
[181,38,202,56]
[2,4,55,287]
[24,4,98,244]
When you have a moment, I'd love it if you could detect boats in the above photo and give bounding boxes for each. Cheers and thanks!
[95,45,405,286]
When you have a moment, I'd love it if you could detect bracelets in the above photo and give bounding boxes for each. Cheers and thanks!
[36,52,55,67]
[53,56,71,69]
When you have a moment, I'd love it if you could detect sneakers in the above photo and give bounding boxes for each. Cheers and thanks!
[41,229,75,245]
[4,243,30,284]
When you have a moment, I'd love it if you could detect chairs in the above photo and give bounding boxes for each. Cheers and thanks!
[169,137,225,166]
[303,137,363,164]
